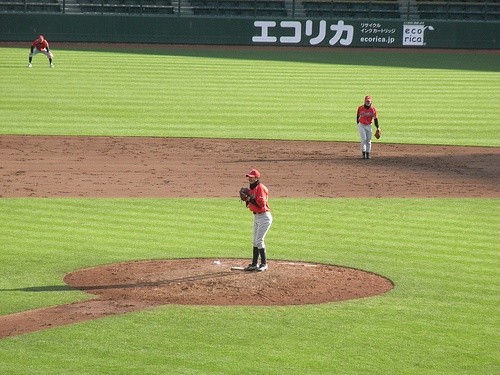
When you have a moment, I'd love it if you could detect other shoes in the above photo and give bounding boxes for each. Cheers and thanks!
[50,65,54,68]
[362,156,371,159]
[29,64,32,67]
[258,263,268,271]
[244,264,259,271]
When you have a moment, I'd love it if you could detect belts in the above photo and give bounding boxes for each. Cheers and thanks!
[253,212,262,215]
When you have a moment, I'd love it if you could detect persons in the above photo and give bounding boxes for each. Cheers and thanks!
[244,169,272,272]
[357,96,380,159]
[28,36,55,68]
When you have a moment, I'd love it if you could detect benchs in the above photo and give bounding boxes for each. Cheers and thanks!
[188,0,287,17]
[304,0,401,18]
[0,0,62,11]
[416,0,500,19]
[78,0,176,15]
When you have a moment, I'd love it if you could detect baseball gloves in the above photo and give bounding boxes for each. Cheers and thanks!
[239,188,249,201]
[374,129,381,139]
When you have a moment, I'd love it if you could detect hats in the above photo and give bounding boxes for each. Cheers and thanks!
[246,169,260,178]
[38,35,43,39]
[365,96,372,102]
